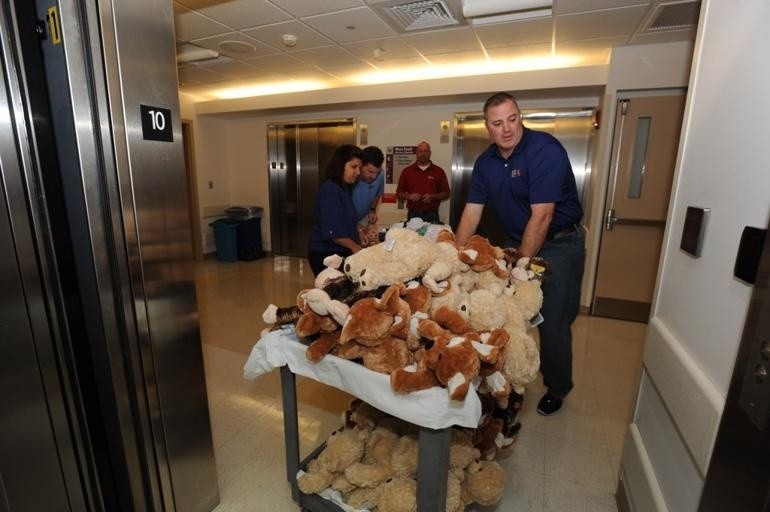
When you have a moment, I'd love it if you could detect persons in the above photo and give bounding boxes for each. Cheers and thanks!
[349,145,388,251]
[395,138,451,222]
[451,89,586,419]
[302,142,367,275]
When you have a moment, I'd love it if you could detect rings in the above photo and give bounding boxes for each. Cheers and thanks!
[358,228,362,232]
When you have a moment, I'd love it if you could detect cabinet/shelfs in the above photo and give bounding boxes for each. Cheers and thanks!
[280,335,453,512]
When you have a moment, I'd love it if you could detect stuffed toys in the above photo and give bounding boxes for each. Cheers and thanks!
[261,217,545,410]
[298,390,524,512]
[259,211,546,512]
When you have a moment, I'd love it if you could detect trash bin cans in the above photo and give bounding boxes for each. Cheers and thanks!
[208,207,264,261]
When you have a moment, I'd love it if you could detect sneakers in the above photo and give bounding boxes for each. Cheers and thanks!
[536,383,574,416]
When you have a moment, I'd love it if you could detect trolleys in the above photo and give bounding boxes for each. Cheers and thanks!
[275,267,536,512]
